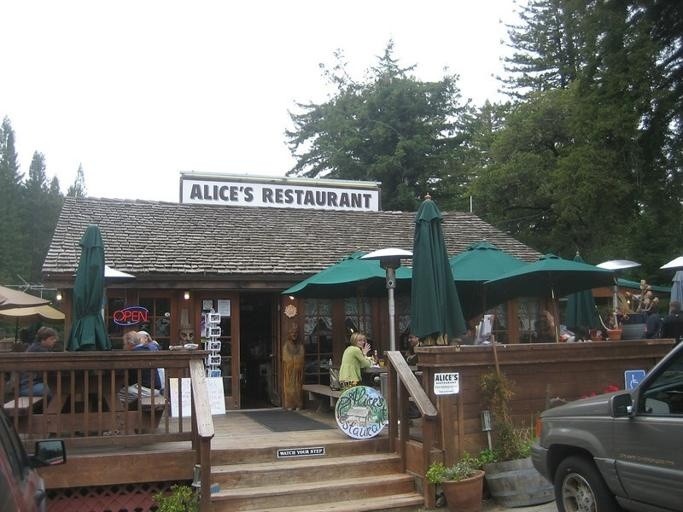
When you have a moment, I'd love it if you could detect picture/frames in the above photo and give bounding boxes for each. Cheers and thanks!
[204,311,223,377]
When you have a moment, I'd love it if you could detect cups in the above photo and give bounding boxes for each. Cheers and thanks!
[378,359,385,369]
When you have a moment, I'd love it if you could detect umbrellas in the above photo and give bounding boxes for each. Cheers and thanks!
[481,251,619,342]
[0,286,50,310]
[409,193,467,345]
[449,239,525,285]
[64,225,111,351]
[0,305,68,343]
[566,250,600,342]
[281,250,412,331]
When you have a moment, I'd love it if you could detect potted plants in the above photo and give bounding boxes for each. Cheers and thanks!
[425,453,485,511]
[479,373,555,509]
[593,304,628,339]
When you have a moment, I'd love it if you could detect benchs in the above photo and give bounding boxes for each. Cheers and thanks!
[2,395,44,417]
[140,394,169,427]
[301,381,346,411]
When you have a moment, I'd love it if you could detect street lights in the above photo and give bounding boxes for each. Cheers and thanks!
[596,260,641,330]
[358,247,415,351]
[659,256,683,302]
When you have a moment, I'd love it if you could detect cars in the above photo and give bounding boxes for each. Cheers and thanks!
[0,406,67,512]
[531,342,683,512]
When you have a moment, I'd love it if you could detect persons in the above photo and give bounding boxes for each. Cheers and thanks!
[138,330,165,393]
[645,303,663,339]
[99,331,161,433]
[336,332,372,390]
[661,301,683,342]
[18,327,58,405]
[404,335,423,366]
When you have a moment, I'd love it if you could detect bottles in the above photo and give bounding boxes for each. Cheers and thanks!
[374,350,378,364]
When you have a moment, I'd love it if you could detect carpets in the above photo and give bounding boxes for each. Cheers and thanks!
[240,408,337,432]
